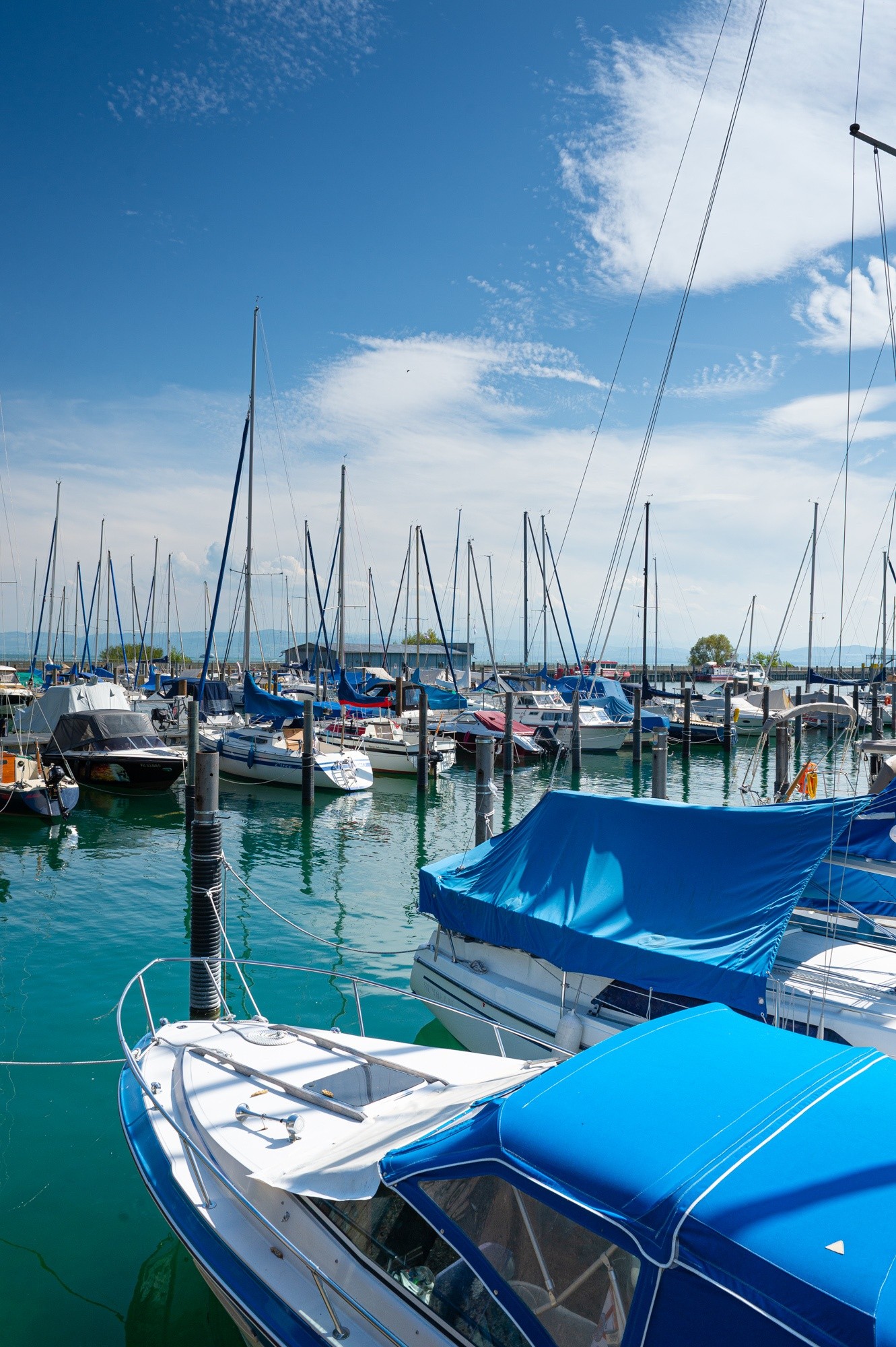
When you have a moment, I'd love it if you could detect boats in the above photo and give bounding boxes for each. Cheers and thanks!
[410,778,896,1060]
[116,956,896,1347]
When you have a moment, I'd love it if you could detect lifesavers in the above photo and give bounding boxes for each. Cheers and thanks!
[797,762,818,793]
[884,694,892,706]
[733,708,740,722]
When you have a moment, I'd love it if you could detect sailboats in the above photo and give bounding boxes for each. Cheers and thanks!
[1,306,896,821]
